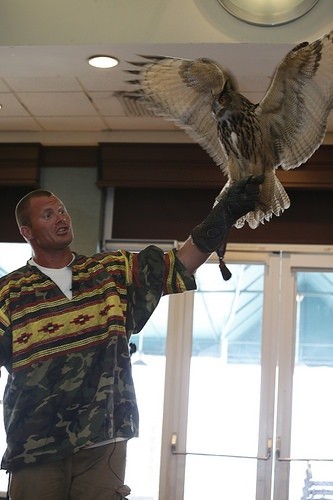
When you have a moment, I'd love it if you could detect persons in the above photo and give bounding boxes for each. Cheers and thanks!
[0,174,271,500]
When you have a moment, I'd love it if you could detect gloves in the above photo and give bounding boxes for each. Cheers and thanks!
[191,173,266,280]
